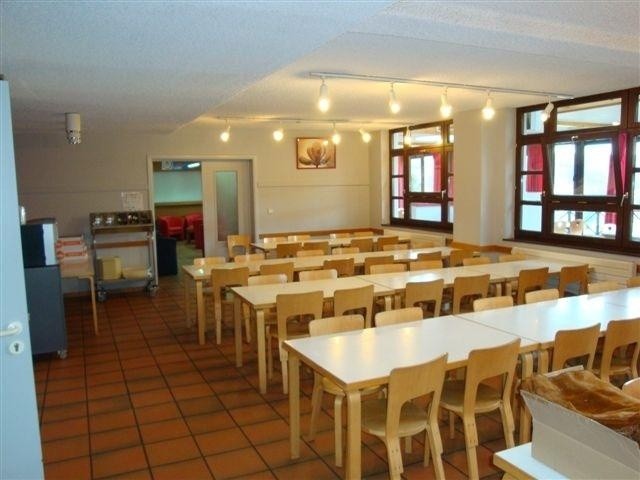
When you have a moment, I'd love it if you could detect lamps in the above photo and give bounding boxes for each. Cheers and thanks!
[482,91,495,119]
[317,77,329,112]
[540,97,554,123]
[439,87,452,116]
[388,82,400,114]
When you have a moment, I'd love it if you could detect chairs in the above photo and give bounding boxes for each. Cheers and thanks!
[453,275,490,313]
[416,252,442,261]
[406,278,445,318]
[463,257,491,266]
[414,242,434,247]
[332,248,359,254]
[354,232,374,236]
[516,267,548,305]
[448,250,472,266]
[551,323,601,371]
[600,318,640,380]
[370,263,407,275]
[329,232,350,239]
[410,260,443,270]
[350,238,373,253]
[378,236,398,251]
[288,235,310,242]
[159,215,185,240]
[267,291,323,378]
[185,214,202,243]
[474,295,512,311]
[194,256,225,264]
[324,258,354,276]
[333,285,375,327]
[299,268,338,281]
[588,281,619,294]
[295,250,324,256]
[374,307,423,327]
[277,242,302,259]
[627,277,640,287]
[382,244,408,250]
[234,255,266,261]
[304,241,329,255]
[259,263,294,281]
[227,235,250,262]
[423,337,521,480]
[360,353,448,479]
[263,237,286,242]
[525,288,559,304]
[558,264,587,298]
[499,254,526,263]
[247,274,288,286]
[307,316,388,469]
[197,267,249,343]
[365,256,395,274]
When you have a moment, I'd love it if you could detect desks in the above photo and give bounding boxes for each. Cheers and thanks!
[229,277,395,394]
[456,285,640,375]
[249,235,412,258]
[357,267,508,309]
[465,258,592,296]
[182,245,462,345]
[60,252,97,347]
[282,314,539,479]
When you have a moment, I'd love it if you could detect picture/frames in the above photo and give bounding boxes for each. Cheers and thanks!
[296,137,336,170]
